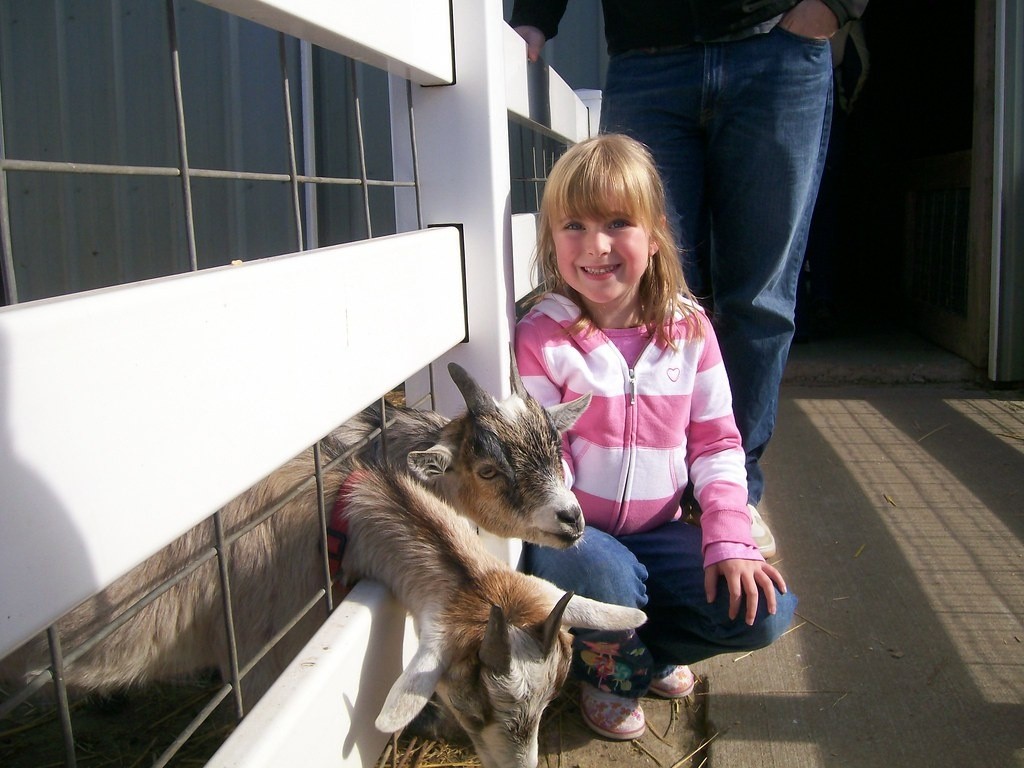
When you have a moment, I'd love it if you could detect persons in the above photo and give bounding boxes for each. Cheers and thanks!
[515,134,799,739]
[509,0,869,559]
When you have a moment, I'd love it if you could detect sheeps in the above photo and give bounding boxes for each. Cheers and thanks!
[0,343,647,768]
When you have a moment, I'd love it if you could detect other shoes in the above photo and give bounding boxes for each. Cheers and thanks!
[647,665,695,699]
[580,681,645,740]
[688,502,777,560]
